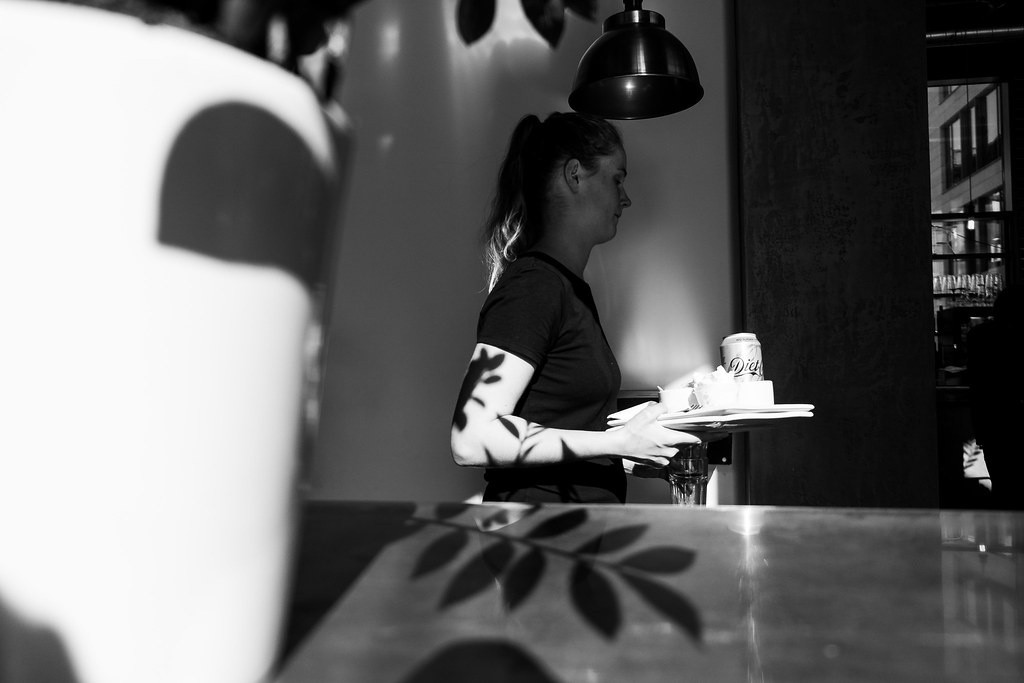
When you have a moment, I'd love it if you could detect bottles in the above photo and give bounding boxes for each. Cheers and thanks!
[934,275,1002,340]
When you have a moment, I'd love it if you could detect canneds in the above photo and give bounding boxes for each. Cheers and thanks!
[720,333,765,382]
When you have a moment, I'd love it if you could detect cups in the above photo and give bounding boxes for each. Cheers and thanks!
[657,372,776,412]
[0,1,333,681]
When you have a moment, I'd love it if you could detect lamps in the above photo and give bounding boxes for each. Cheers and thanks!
[569,0,705,122]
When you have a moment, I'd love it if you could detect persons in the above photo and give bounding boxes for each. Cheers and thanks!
[450,112,730,505]
